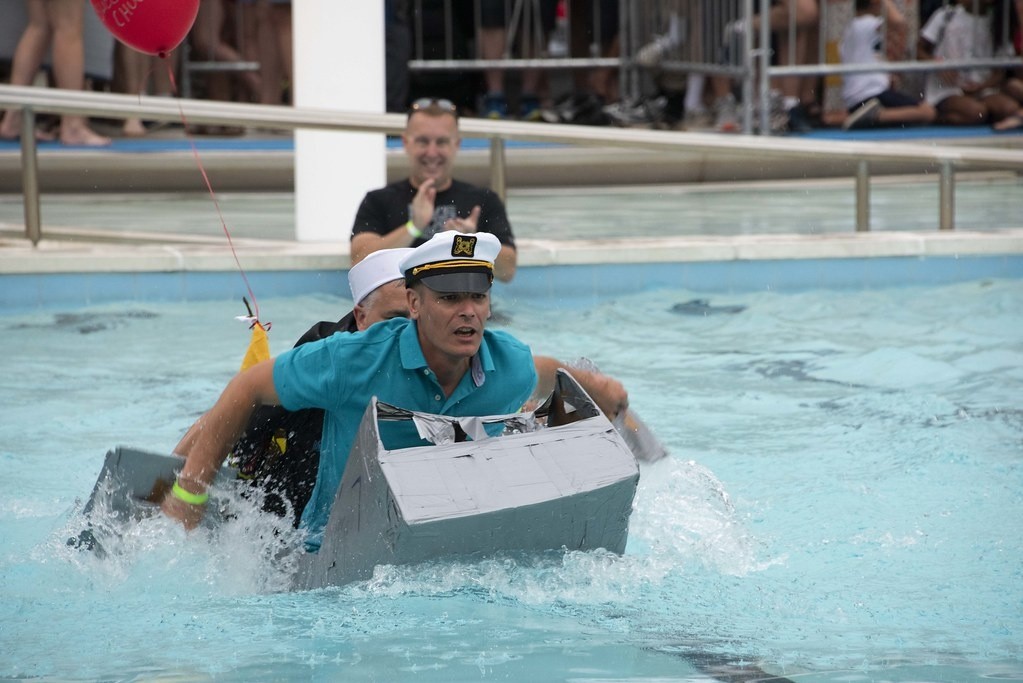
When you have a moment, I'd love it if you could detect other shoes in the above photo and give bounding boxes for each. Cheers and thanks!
[484,92,635,129]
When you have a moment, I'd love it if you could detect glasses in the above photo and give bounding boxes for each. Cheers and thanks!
[409,98,456,114]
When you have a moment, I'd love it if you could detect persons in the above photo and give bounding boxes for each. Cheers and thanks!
[161,229,629,555]
[477,0,1023,135]
[350,97,517,283]
[0,0,292,145]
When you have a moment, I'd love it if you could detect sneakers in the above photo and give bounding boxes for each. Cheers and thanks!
[844,98,882,132]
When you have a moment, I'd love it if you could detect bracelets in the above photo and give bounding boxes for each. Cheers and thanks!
[405,220,423,238]
[172,478,208,505]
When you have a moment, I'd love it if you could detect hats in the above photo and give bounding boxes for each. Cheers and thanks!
[348,248,416,305]
[399,229,502,294]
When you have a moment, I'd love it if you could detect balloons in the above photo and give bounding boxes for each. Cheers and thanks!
[91,0,200,58]
